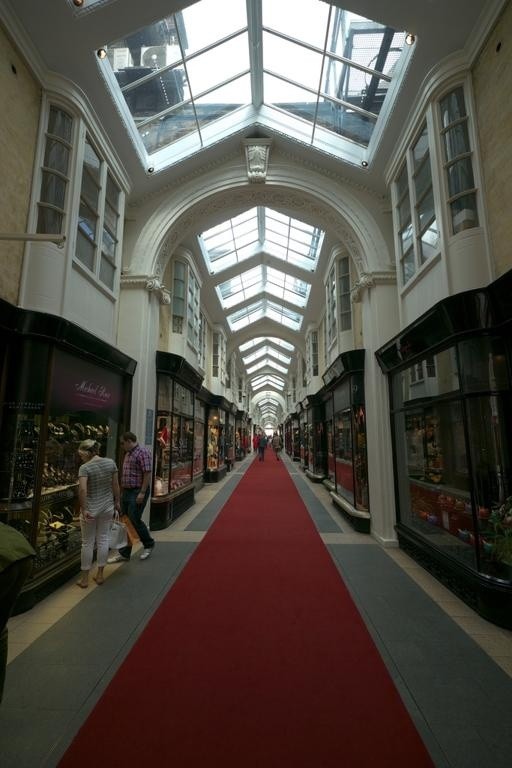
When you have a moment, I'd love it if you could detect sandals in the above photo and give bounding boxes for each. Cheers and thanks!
[76,574,105,589]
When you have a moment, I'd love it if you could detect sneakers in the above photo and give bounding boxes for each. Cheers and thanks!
[107,552,133,564]
[138,546,154,560]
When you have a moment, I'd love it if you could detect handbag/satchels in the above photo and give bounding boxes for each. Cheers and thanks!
[108,510,129,549]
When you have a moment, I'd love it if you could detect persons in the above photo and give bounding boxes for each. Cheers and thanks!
[0,520,38,704]
[253,434,273,449]
[258,436,267,464]
[157,417,168,477]
[108,430,154,564]
[272,434,283,461]
[73,438,120,587]
